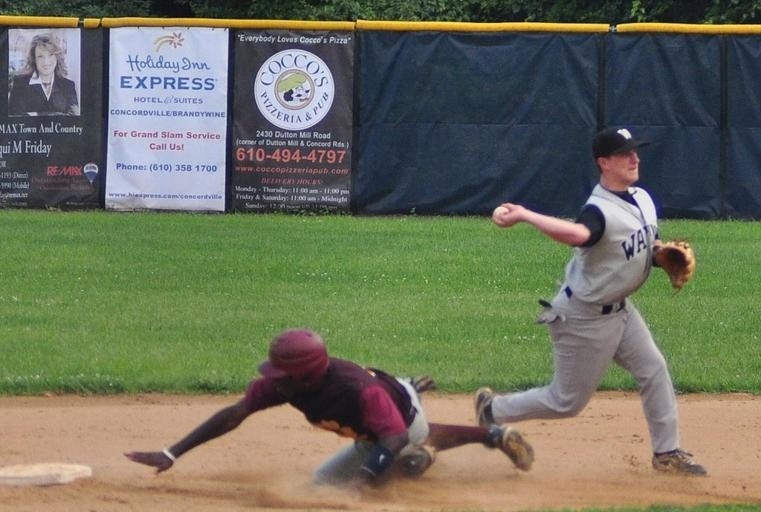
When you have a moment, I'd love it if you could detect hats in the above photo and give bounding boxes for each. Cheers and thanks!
[591,127,649,156]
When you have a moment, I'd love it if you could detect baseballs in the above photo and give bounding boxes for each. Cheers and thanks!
[493,206,509,216]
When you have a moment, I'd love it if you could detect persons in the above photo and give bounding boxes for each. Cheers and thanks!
[123,330,534,500]
[7,33,79,118]
[474,125,708,480]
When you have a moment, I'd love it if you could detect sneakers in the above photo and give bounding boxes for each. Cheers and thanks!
[474,387,494,425]
[409,445,436,479]
[485,425,534,471]
[653,450,706,476]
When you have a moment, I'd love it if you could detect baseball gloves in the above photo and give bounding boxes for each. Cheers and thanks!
[653,241,696,288]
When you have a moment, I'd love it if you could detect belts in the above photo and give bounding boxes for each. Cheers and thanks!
[564,287,624,314]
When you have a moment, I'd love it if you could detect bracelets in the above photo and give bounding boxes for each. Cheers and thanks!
[162,447,176,464]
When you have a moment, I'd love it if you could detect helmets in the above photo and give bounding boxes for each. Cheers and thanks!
[258,330,330,383]
